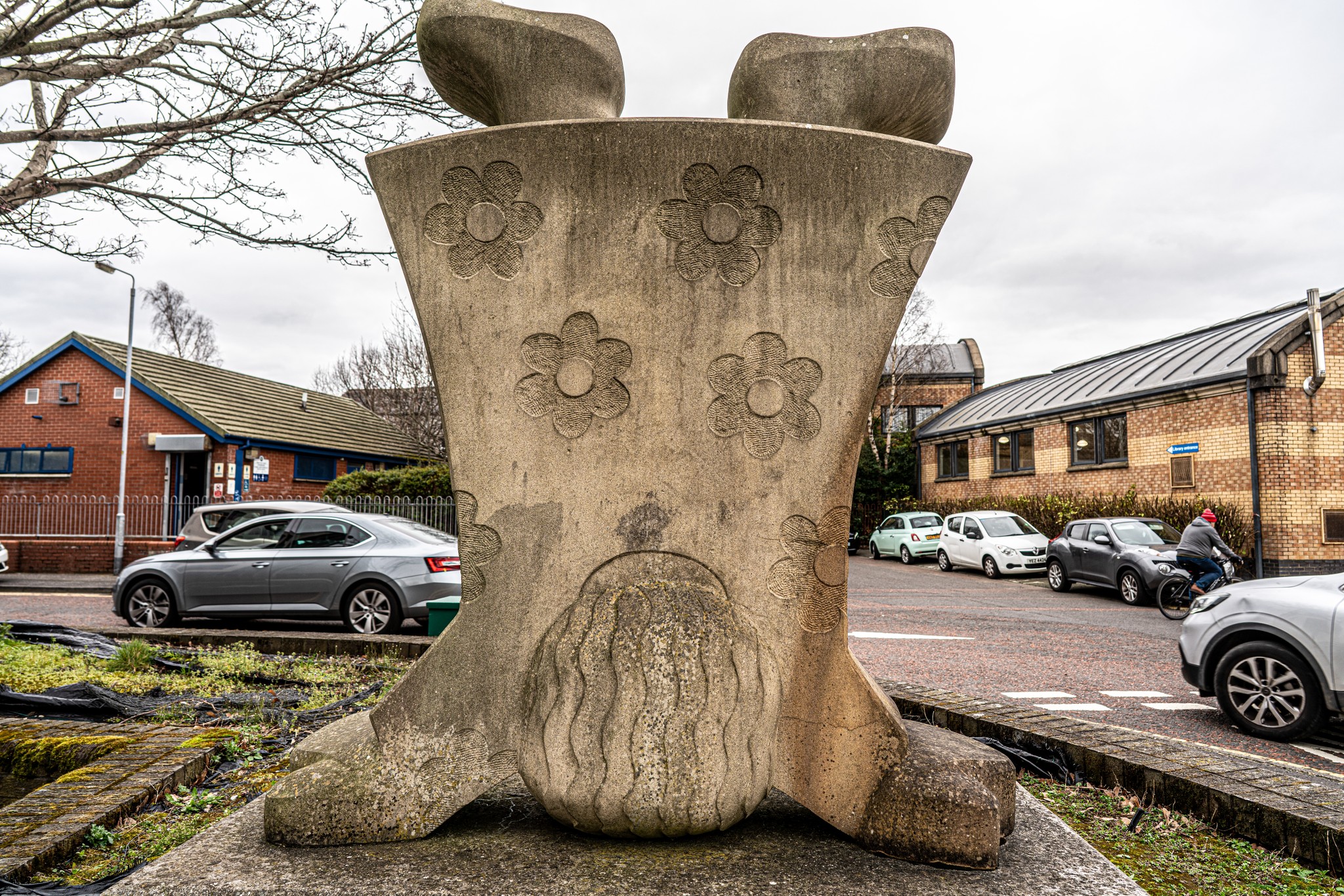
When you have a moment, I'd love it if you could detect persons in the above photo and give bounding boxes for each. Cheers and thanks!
[1176,508,1242,605]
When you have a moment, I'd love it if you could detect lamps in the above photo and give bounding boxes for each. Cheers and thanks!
[1076,426,1079,432]
[1076,424,1088,447]
[998,436,1009,444]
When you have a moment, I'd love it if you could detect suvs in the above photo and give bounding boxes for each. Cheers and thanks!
[173,499,358,552]
[1177,569,1344,741]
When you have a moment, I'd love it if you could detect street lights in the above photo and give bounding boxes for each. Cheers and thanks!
[94,262,136,578]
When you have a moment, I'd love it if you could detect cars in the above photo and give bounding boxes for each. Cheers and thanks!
[112,513,463,634]
[1046,516,1236,607]
[935,510,1050,579]
[869,511,944,565]
[847,530,860,556]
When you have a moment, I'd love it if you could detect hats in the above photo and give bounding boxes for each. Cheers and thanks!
[1201,508,1217,522]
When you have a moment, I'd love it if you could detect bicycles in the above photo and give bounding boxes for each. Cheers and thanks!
[1157,557,1245,621]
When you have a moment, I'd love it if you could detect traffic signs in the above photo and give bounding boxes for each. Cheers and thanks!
[1166,443,1199,454]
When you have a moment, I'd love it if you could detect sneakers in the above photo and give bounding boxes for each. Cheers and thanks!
[1190,584,1206,595]
